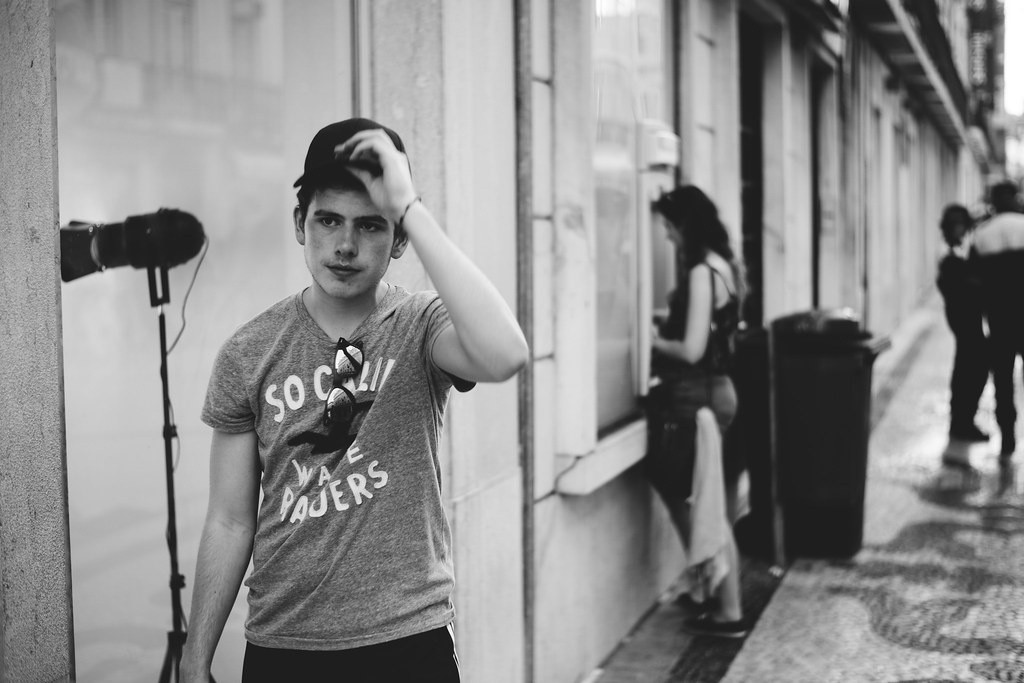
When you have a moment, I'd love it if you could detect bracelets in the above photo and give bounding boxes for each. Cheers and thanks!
[399,196,422,224]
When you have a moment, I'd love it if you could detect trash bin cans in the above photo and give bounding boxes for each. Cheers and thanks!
[736,310,891,561]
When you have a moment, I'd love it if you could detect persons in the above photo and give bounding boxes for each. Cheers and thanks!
[651,185,750,638]
[178,119,530,683]
[935,182,1024,457]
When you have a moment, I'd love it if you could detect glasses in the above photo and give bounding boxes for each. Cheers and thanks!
[321,337,364,429]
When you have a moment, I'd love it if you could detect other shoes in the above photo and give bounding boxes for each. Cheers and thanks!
[999,440,1016,456]
[682,613,745,638]
[949,425,989,442]
[677,591,711,619]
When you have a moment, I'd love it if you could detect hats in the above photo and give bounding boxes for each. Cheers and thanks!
[293,118,409,186]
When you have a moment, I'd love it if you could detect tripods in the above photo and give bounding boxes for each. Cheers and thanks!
[160,304,218,683]
